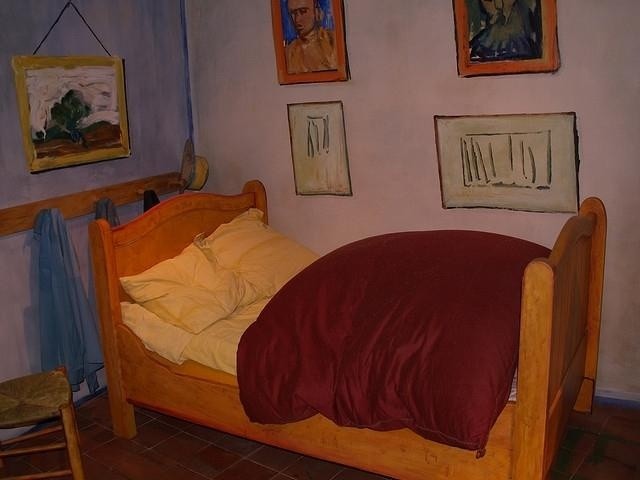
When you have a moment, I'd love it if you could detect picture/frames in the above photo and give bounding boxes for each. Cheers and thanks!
[451,0,560,78]
[10,55,130,175]
[271,0,349,86]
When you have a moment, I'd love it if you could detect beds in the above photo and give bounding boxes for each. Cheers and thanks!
[85,180,610,479]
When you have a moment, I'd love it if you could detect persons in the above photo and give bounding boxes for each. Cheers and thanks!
[284,0,338,74]
[468,0,543,60]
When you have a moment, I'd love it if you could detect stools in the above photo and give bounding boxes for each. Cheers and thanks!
[1,367,84,479]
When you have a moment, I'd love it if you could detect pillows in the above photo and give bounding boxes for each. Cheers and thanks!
[117,207,323,334]
